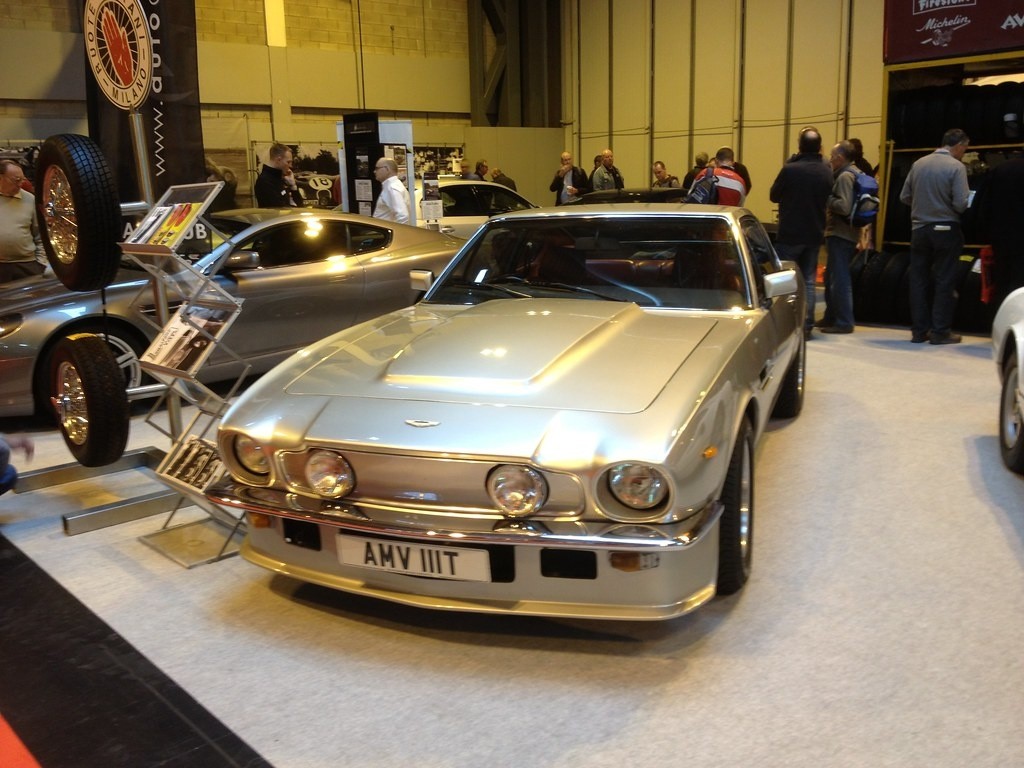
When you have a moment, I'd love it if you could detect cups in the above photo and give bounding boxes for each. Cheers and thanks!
[567,186,573,195]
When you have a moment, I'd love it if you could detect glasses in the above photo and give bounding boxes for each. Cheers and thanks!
[3,174,27,185]
[374,166,384,170]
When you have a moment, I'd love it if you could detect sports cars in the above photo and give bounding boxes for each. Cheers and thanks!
[0,206,468,428]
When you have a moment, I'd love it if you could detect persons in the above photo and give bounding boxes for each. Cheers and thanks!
[372,158,411,225]
[0,159,52,285]
[491,168,518,208]
[468,160,489,189]
[588,155,603,184]
[770,125,830,339]
[254,143,304,208]
[683,148,752,207]
[204,173,238,216]
[815,139,873,334]
[592,149,624,191]
[652,161,681,188]
[899,128,970,345]
[550,151,590,206]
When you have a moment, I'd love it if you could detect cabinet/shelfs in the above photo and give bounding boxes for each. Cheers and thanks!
[117,181,254,569]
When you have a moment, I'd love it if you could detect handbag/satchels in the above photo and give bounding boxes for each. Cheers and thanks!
[834,167,880,228]
[686,166,719,205]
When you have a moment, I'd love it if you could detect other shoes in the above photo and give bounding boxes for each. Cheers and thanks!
[804,332,814,341]
[819,325,853,333]
[929,333,962,345]
[812,319,833,327]
[910,332,934,343]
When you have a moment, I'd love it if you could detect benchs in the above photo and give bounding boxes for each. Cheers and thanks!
[524,259,739,290]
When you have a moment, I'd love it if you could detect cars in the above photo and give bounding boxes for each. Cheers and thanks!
[204,203,809,624]
[992,287,1023,476]
[560,187,701,205]
[369,180,545,242]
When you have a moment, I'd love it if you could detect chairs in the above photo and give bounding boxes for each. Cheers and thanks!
[529,246,588,293]
[672,241,727,291]
